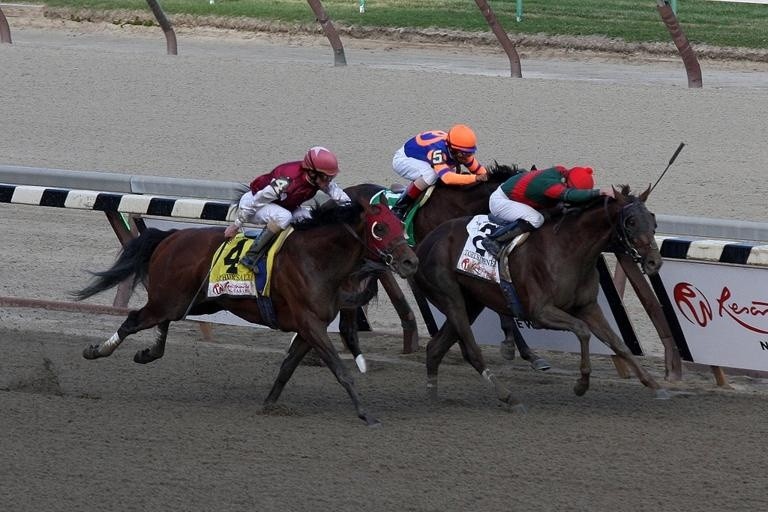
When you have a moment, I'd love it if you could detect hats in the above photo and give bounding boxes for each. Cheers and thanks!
[568,165,594,190]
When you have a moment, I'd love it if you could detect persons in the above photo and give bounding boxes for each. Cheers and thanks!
[482,164,615,259]
[389,123,488,220]
[223,144,350,272]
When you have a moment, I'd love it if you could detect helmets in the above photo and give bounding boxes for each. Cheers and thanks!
[302,145,341,177]
[446,123,477,154]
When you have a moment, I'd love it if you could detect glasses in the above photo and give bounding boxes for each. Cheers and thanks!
[310,169,336,182]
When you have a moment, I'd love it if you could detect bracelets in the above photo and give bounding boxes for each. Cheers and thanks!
[233,218,242,228]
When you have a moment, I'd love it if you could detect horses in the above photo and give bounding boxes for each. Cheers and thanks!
[62,192,419,429]
[352,183,672,408]
[338,159,552,367]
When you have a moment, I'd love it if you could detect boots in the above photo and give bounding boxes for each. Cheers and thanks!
[240,221,285,273]
[391,180,423,221]
[481,216,537,260]
